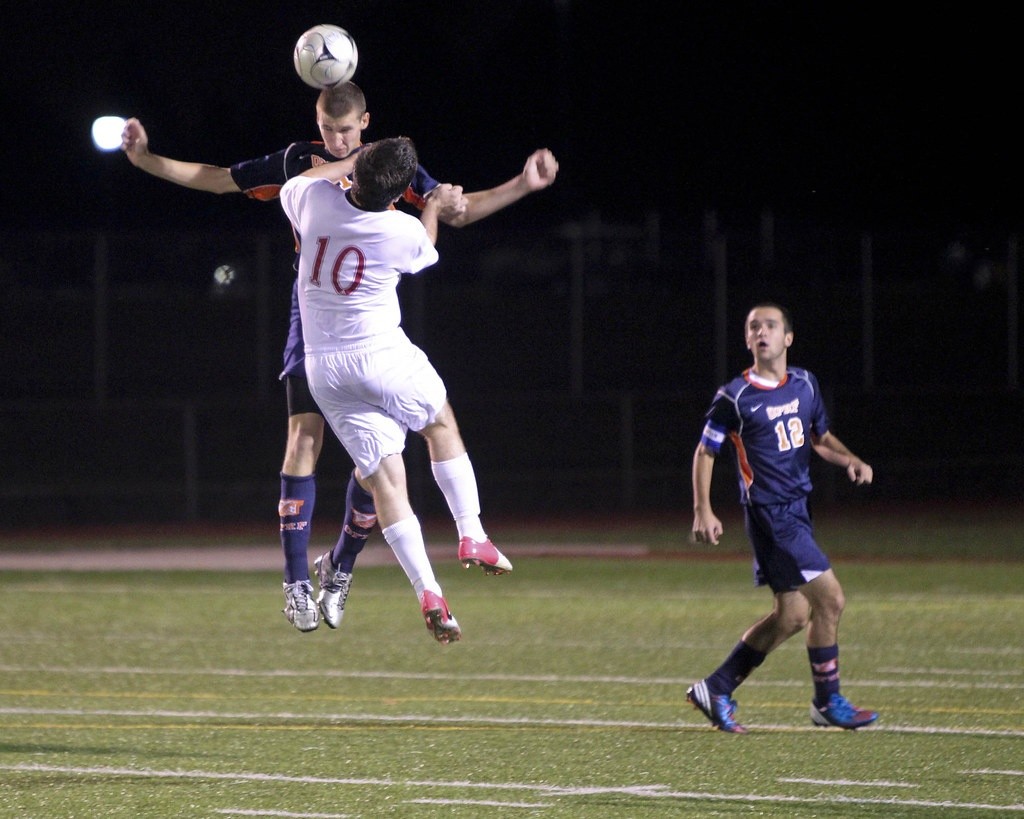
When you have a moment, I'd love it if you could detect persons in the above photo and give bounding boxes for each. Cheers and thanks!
[121,80,560,633]
[685,302,878,734]
[279,136,512,644]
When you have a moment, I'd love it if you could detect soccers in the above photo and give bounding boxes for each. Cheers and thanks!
[292,23,359,91]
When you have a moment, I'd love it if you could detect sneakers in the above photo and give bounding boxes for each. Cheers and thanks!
[457,535,514,577]
[314,550,354,629]
[419,588,463,645]
[281,578,321,632]
[685,678,747,733]
[810,692,879,729]
